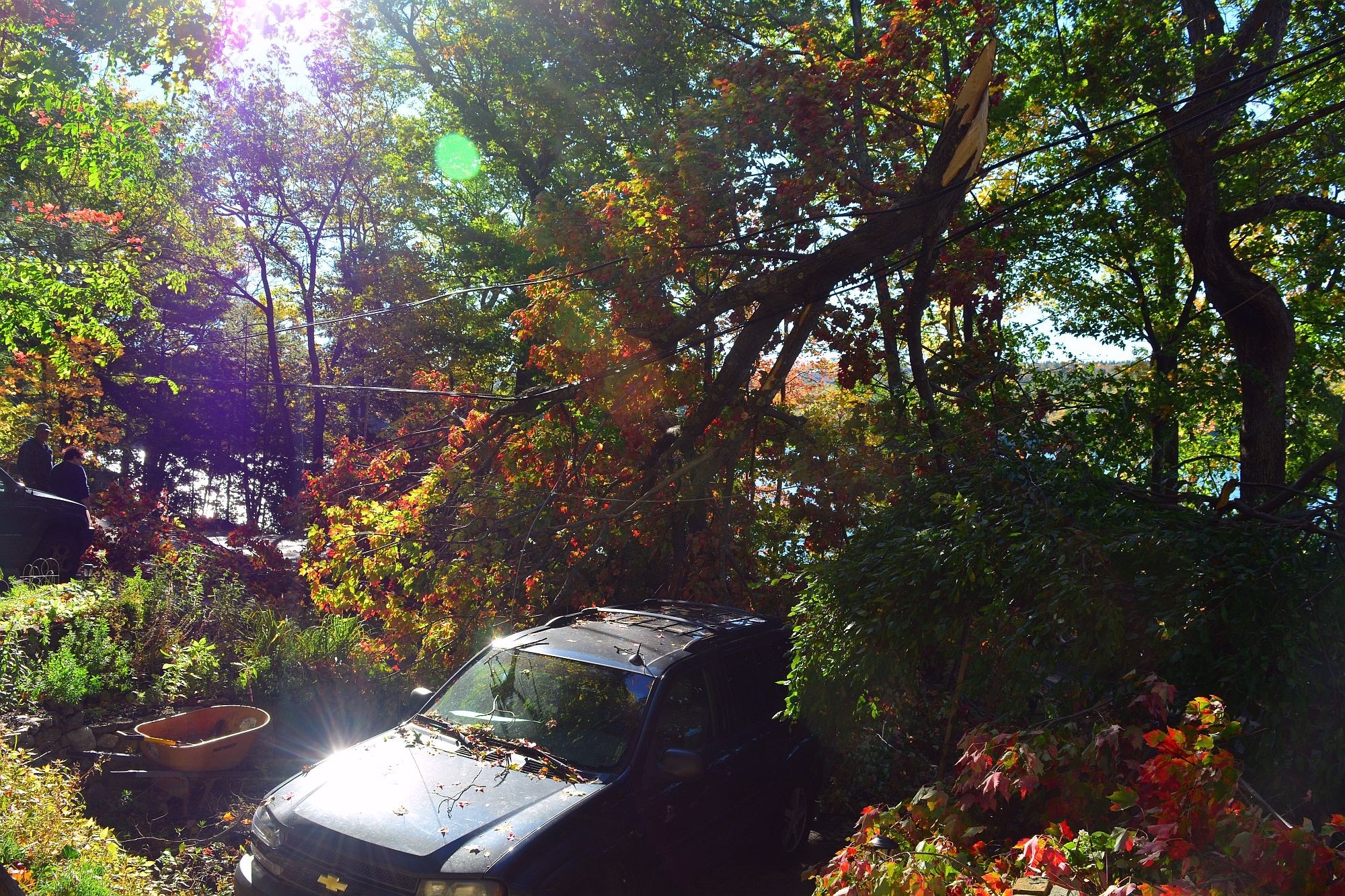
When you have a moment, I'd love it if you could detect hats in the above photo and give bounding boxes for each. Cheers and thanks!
[36,423,52,433]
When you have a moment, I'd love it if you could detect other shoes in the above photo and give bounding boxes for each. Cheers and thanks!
[76,567,88,578]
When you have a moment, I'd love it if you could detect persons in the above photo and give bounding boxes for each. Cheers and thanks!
[47,444,90,510]
[13,421,53,495]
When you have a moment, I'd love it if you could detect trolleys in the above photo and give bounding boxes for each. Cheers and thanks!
[81,701,271,776]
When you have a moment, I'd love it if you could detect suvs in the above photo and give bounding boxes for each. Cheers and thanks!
[232,594,810,896]
